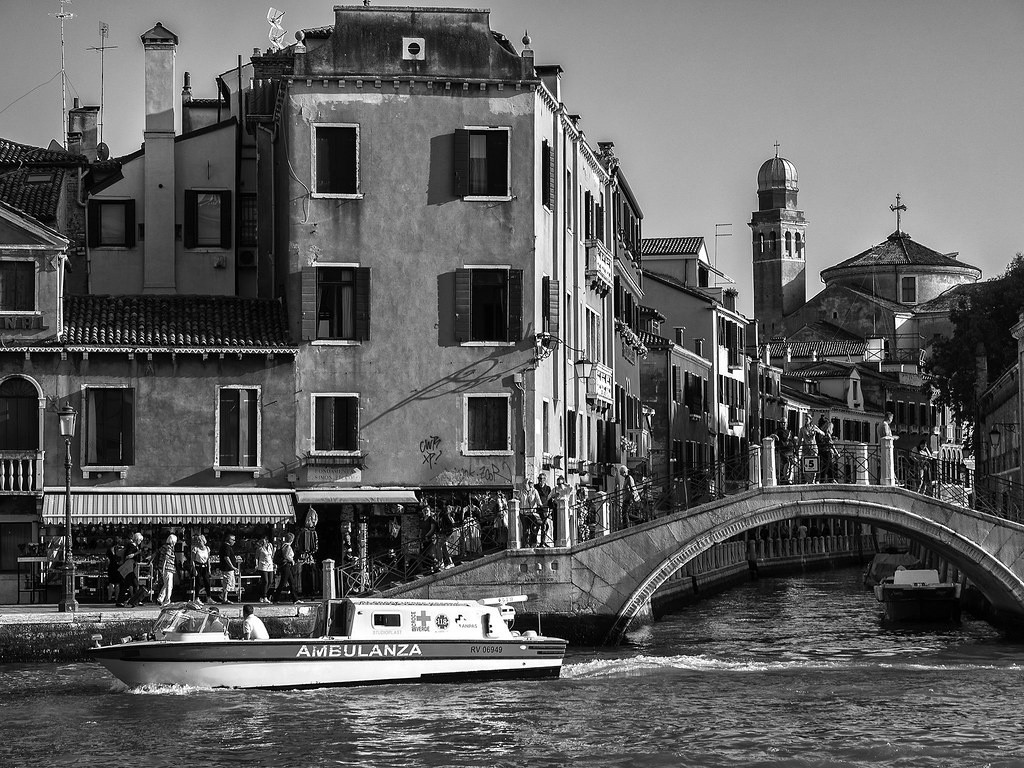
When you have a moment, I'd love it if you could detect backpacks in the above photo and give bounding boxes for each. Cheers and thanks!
[273,544,289,565]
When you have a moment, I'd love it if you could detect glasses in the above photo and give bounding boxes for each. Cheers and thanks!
[537,477,545,480]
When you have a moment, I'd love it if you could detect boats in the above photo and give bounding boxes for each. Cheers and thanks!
[874,565,961,631]
[87,594,569,691]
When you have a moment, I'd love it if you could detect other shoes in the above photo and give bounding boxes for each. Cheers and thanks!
[218,594,233,605]
[833,479,838,484]
[524,544,537,548]
[805,481,808,485]
[895,481,905,487]
[540,542,550,548]
[812,479,815,484]
[293,600,305,605]
[438,562,444,570]
[444,563,455,570]
[816,480,820,484]
[635,518,645,525]
[124,603,134,608]
[116,602,124,607]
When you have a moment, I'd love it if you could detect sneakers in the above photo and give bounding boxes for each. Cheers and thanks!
[259,596,271,603]
[206,597,217,604]
[194,597,205,606]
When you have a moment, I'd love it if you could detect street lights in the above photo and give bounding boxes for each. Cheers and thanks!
[57,400,79,612]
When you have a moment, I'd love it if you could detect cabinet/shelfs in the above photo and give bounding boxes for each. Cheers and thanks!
[15,556,49,603]
[134,562,154,603]
[192,558,243,602]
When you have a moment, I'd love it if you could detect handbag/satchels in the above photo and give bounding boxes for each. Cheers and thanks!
[548,497,557,509]
[633,492,641,503]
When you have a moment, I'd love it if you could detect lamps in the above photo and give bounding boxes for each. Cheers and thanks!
[988,421,1019,451]
[537,333,593,384]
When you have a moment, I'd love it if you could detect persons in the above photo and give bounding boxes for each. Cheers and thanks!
[515,473,572,548]
[770,415,840,484]
[106,533,143,606]
[760,520,864,558]
[619,465,645,530]
[254,533,304,604]
[191,535,217,606]
[220,535,240,605]
[909,440,932,494]
[146,534,177,605]
[240,604,269,641]
[419,490,508,576]
[196,607,228,632]
[878,412,906,487]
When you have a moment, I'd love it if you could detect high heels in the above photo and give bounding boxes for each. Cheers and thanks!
[156,599,162,606]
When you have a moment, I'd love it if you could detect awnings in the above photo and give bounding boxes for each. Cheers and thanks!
[295,490,418,503]
[39,494,296,526]
